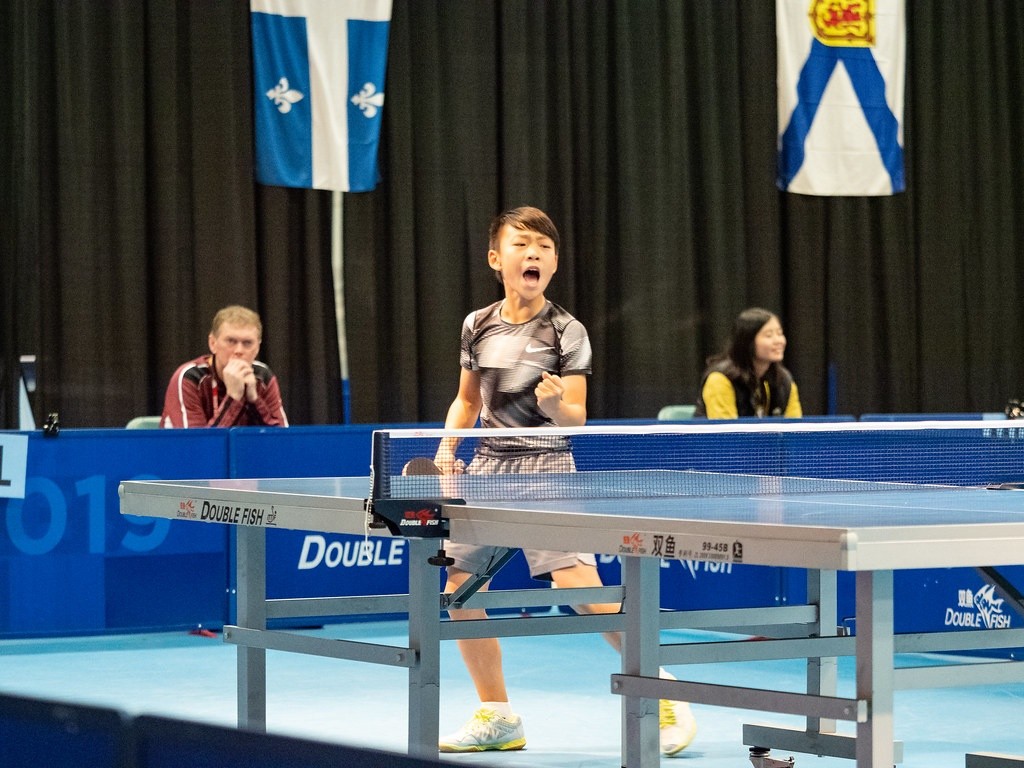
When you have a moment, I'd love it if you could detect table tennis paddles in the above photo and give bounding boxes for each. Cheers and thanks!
[401,457,467,476]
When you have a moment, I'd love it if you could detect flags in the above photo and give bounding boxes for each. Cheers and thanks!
[774,0,906,197]
[250,0,392,194]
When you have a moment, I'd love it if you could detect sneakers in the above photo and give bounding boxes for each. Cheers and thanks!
[659,672,697,755]
[438,707,527,753]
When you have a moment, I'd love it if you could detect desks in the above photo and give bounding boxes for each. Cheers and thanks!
[118,470,1023,768]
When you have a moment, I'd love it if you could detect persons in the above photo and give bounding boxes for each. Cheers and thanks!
[433,208,697,755]
[160,306,290,428]
[693,307,803,420]
